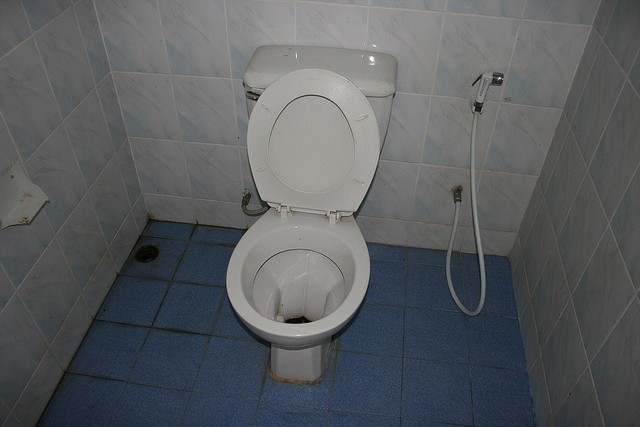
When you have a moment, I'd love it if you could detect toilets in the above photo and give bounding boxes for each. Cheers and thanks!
[226,45,398,382]
